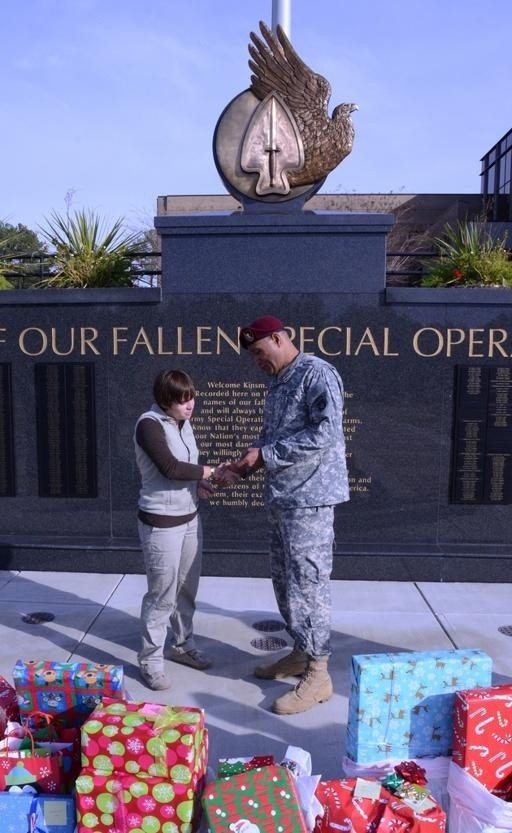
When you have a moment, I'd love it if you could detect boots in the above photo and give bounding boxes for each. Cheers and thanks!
[255,643,311,680]
[272,655,334,715]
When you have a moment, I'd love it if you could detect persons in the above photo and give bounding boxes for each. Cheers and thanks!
[132,368,226,691]
[227,317,351,713]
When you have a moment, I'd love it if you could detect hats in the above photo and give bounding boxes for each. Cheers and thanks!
[240,315,285,350]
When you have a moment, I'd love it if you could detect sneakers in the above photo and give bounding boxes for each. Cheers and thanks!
[141,671,172,690]
[171,648,212,670]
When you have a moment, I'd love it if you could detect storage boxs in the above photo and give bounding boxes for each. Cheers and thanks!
[312,774,447,832]
[74,729,210,832]
[79,695,207,781]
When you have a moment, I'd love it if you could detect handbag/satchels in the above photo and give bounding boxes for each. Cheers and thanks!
[1,711,81,794]
